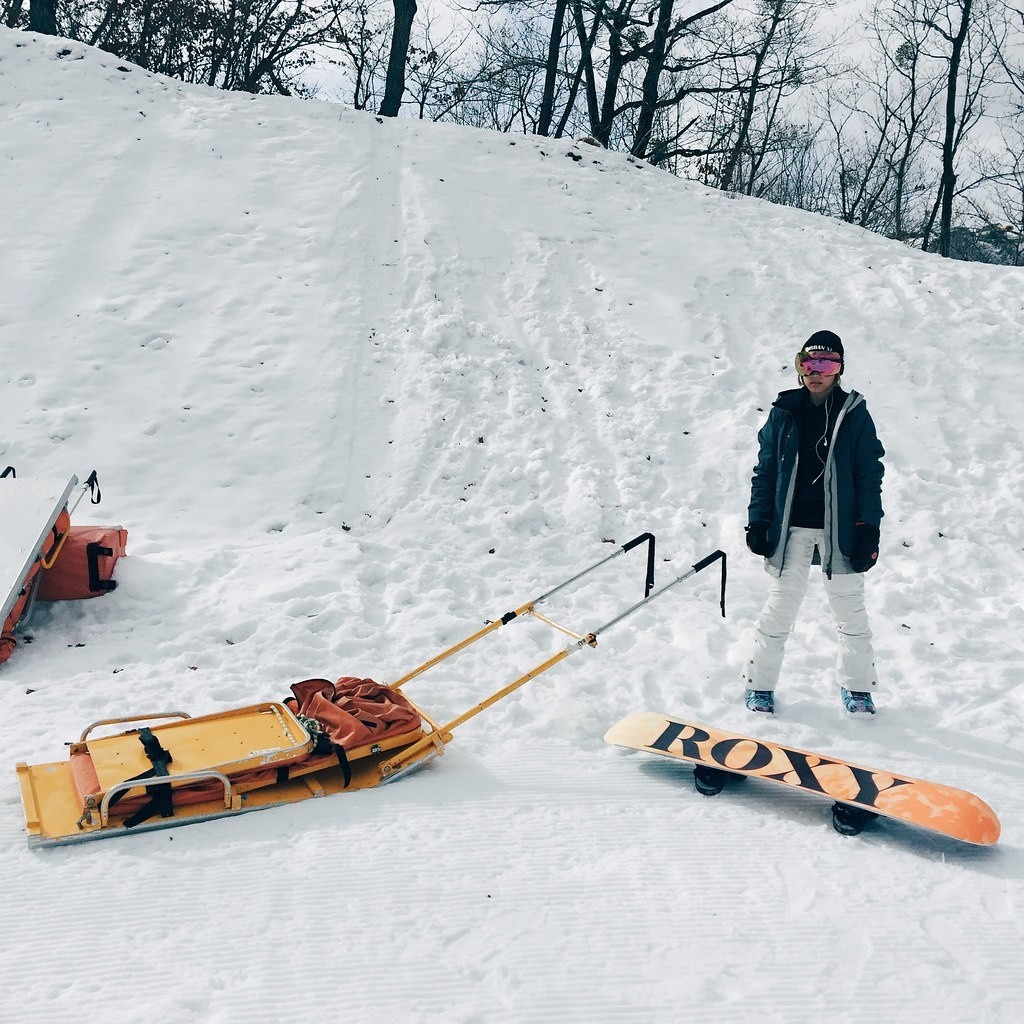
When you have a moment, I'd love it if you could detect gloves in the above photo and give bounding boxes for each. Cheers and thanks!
[849,525,880,572]
[745,521,777,558]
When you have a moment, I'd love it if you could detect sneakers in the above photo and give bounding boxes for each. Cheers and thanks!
[745,689,774,715]
[840,686,876,714]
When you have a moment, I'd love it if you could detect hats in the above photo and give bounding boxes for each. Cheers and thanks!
[800,330,844,375]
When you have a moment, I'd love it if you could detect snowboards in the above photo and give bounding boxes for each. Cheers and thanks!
[601,709,1004,848]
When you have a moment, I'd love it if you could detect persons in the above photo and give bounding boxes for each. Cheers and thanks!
[745,330,886,715]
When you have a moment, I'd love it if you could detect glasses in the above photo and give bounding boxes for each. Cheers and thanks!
[794,352,842,376]
